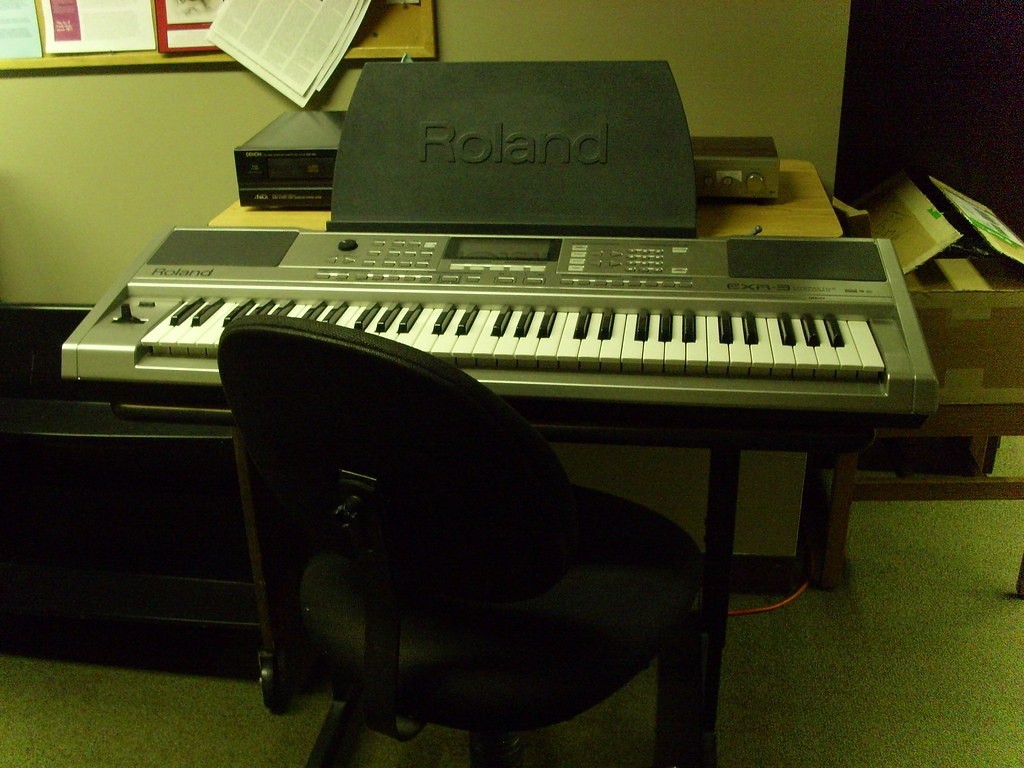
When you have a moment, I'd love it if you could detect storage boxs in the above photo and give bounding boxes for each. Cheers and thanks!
[855,168,1024,404]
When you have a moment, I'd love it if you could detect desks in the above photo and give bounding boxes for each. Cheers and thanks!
[112,393,874,713]
[205,159,843,238]
[818,404,1024,599]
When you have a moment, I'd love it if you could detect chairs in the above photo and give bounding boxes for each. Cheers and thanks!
[219,320,706,768]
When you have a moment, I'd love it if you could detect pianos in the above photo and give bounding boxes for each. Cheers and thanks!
[60,225,942,418]
